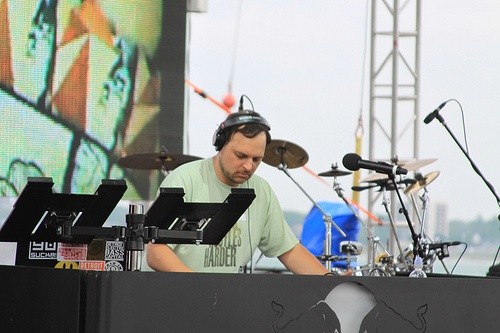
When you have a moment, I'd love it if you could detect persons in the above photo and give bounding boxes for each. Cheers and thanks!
[146,110,352,276]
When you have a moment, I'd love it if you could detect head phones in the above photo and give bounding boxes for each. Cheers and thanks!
[212,115,272,152]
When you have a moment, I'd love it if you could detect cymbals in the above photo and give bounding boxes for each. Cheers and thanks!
[318,171,353,177]
[360,157,439,182]
[262,140,309,169]
[403,170,440,195]
[118,153,204,170]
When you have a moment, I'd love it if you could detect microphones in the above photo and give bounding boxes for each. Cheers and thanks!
[342,153,407,175]
[424,102,446,124]
[423,241,462,250]
[239,96,244,110]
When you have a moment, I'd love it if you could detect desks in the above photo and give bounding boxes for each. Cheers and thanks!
[1,262,500,333]
